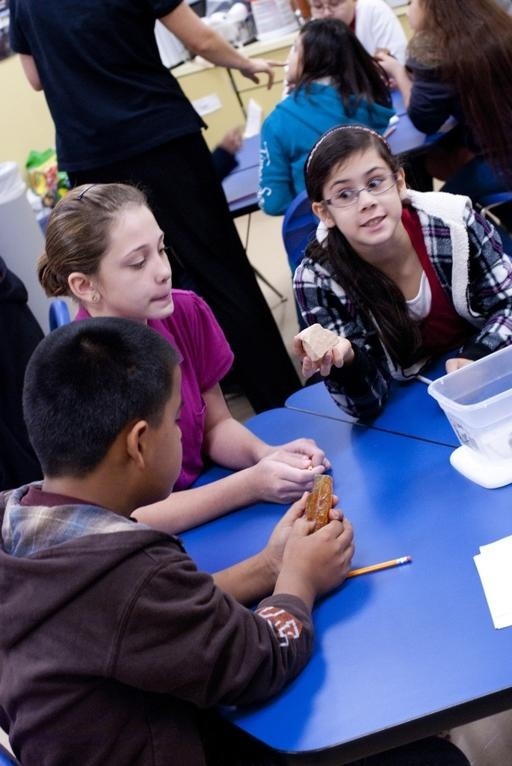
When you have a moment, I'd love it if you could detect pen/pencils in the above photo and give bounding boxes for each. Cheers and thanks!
[416,374,433,384]
[345,555,412,578]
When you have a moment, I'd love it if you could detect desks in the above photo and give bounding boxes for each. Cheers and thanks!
[87,71,512,307]
[153,316,512,763]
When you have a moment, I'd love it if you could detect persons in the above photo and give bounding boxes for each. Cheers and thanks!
[7,0,304,423]
[0,311,358,763]
[257,16,396,217]
[284,1,407,99]
[36,181,334,538]
[290,122,512,426]
[376,0,511,197]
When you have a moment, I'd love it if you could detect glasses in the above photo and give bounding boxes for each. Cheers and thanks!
[321,174,398,209]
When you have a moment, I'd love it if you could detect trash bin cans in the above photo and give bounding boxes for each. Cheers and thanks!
[0,162,47,279]
